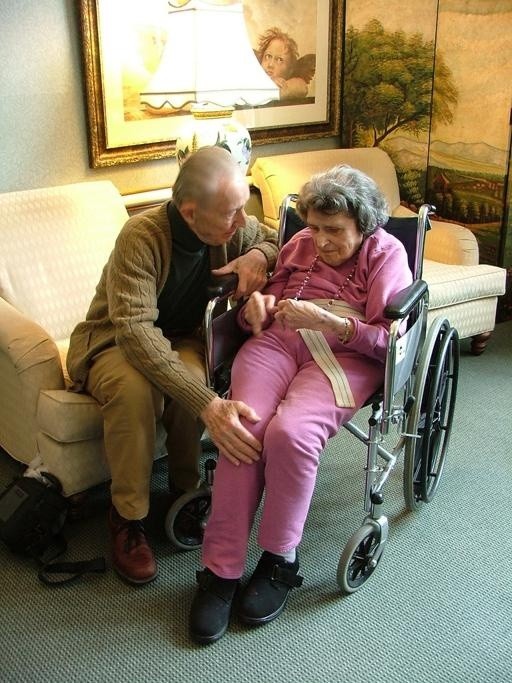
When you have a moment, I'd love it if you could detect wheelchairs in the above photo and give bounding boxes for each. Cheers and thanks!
[164,193,461,594]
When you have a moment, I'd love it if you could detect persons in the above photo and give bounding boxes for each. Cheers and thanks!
[187,163,414,645]
[63,145,280,586]
[227,25,308,106]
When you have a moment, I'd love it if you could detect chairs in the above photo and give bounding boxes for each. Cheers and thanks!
[0,180,210,520]
[250,147,507,354]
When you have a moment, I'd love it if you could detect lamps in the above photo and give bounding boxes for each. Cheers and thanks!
[139,5,281,169]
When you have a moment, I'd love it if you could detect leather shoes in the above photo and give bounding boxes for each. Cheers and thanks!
[108,505,159,585]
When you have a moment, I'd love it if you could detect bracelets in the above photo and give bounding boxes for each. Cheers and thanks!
[340,318,351,343]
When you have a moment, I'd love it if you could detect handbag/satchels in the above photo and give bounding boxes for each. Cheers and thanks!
[1,471,71,554]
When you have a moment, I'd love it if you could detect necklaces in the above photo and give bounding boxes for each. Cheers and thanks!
[288,244,360,311]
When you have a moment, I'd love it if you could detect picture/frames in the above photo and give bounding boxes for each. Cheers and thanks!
[82,0,346,168]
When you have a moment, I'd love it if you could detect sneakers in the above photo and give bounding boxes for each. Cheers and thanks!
[234,550,303,626]
[188,568,240,643]
